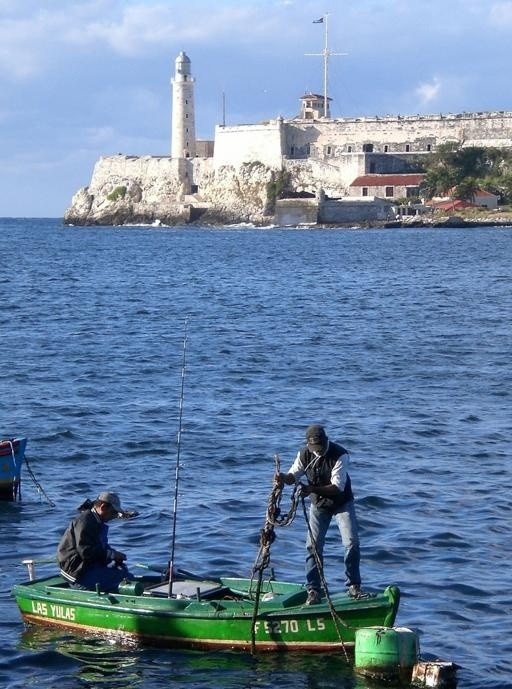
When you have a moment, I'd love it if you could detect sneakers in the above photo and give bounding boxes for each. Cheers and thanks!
[345,584,377,601]
[305,589,321,606]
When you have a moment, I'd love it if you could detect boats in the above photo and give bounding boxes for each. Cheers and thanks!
[0,432,31,503]
[7,557,404,662]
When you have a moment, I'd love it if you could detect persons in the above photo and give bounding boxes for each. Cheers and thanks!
[274,424,362,606]
[55,491,131,594]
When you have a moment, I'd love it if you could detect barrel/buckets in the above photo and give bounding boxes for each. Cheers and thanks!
[398,625,420,666]
[354,626,396,669]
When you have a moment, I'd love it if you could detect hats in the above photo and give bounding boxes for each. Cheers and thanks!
[306,426,326,452]
[98,492,125,514]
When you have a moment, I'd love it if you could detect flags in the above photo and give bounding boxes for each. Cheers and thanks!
[312,16,323,24]
